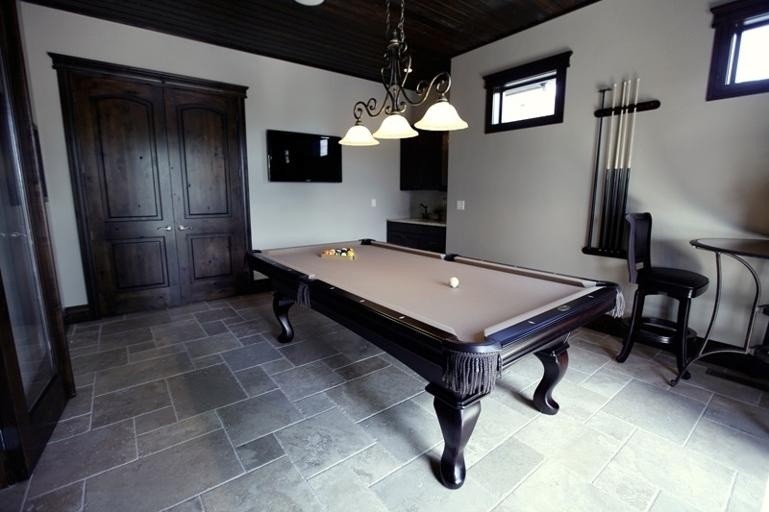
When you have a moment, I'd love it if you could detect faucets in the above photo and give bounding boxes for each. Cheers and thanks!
[419,203,428,216]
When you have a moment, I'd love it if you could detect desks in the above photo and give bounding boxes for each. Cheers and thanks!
[385,221,446,256]
[243,236,622,491]
[670,234,768,417]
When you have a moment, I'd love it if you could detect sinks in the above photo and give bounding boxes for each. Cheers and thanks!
[406,218,431,222]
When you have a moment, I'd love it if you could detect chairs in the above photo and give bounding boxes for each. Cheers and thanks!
[613,210,709,384]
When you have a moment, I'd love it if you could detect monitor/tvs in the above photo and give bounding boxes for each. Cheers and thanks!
[267,130,341,182]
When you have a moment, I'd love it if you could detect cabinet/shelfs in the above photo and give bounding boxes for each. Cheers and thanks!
[398,120,446,190]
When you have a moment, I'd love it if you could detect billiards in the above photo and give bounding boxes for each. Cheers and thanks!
[321,248,354,256]
[449,276,459,288]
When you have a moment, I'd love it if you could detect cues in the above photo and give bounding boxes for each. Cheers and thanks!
[587,79,640,254]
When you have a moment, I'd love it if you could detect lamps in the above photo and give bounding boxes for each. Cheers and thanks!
[336,1,468,150]
